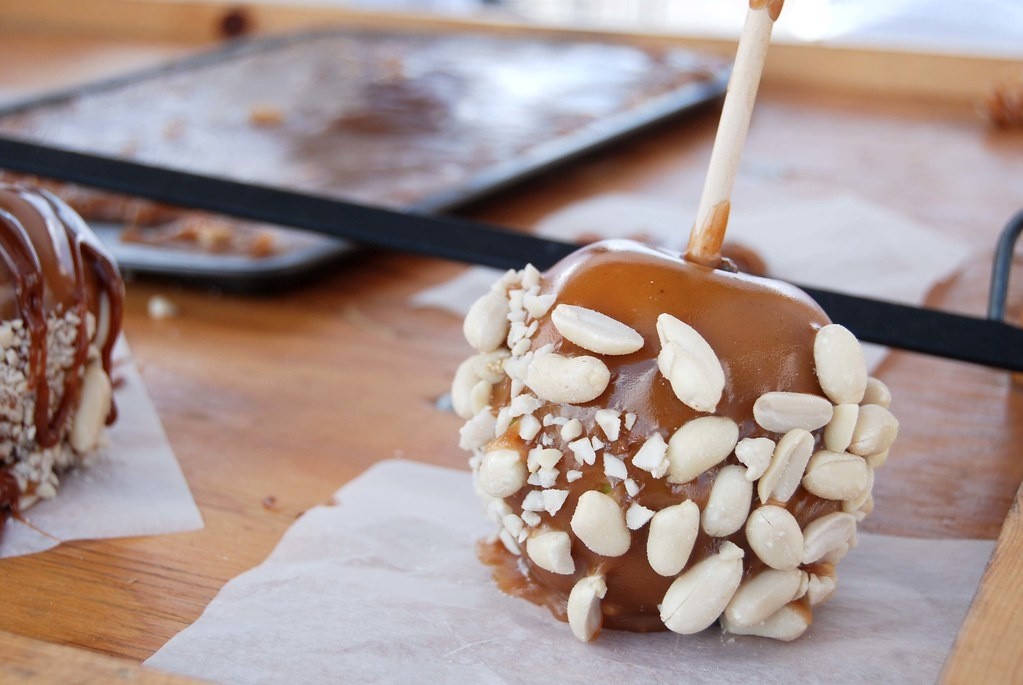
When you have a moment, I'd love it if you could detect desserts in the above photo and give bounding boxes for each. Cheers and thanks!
[444,238,904,644]
[0,177,126,525]
